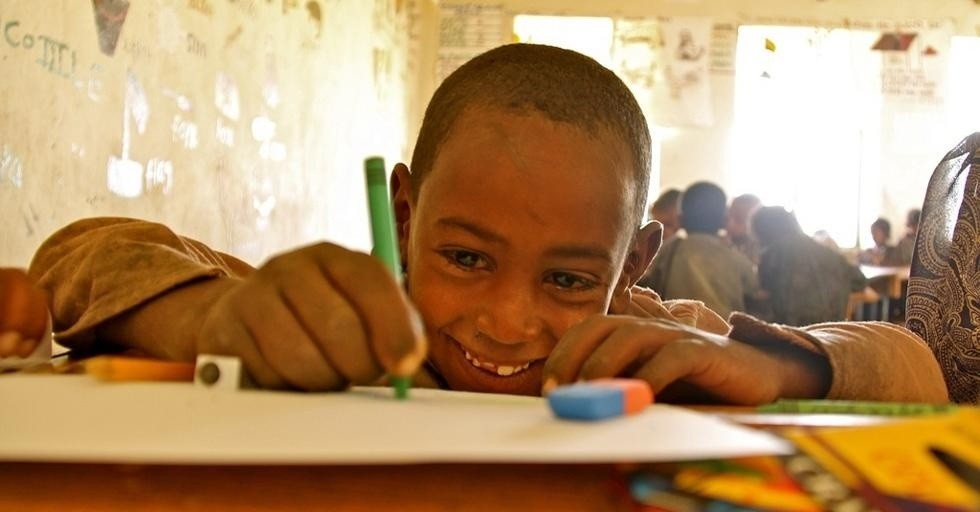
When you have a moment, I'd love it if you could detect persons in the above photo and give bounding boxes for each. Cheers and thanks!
[645,187,684,241]
[857,214,905,267]
[810,229,841,251]
[741,205,869,325]
[895,204,922,267]
[28,44,952,405]
[637,178,761,319]
[726,192,761,261]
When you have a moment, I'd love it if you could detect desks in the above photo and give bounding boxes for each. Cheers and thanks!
[0,370,980,512]
[743,262,911,322]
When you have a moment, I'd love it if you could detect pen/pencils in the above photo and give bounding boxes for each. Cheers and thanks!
[364,157,411,399]
[69,355,195,382]
[755,398,959,415]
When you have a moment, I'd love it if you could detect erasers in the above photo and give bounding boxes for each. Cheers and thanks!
[548,377,653,420]
